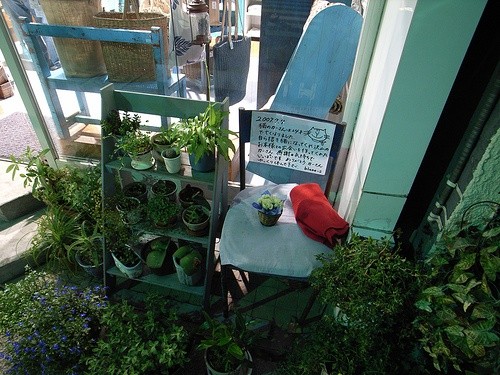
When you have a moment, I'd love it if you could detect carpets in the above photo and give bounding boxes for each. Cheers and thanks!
[0,112,77,166]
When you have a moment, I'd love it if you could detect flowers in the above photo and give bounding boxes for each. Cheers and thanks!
[251,185,287,216]
[0,264,111,375]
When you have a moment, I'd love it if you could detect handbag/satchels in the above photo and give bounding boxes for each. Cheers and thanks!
[212,0,252,106]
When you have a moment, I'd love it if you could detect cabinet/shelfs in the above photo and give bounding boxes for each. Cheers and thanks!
[101,83,228,311]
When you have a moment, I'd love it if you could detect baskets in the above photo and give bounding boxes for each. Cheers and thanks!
[94,0,171,84]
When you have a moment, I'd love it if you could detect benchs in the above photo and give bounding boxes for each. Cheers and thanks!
[18,15,188,144]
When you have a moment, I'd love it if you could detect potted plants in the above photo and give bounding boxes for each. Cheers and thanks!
[108,130,156,176]
[178,183,204,209]
[107,194,142,221]
[122,181,148,204]
[171,100,240,172]
[193,308,262,375]
[145,193,179,228]
[181,204,210,232]
[71,171,144,279]
[149,178,178,202]
[172,246,202,286]
[63,220,104,276]
[160,137,182,174]
[141,235,173,276]
[151,128,179,161]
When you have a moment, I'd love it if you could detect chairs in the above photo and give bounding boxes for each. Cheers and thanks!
[219,108,350,329]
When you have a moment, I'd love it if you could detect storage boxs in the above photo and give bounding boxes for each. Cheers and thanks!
[0,66,14,99]
